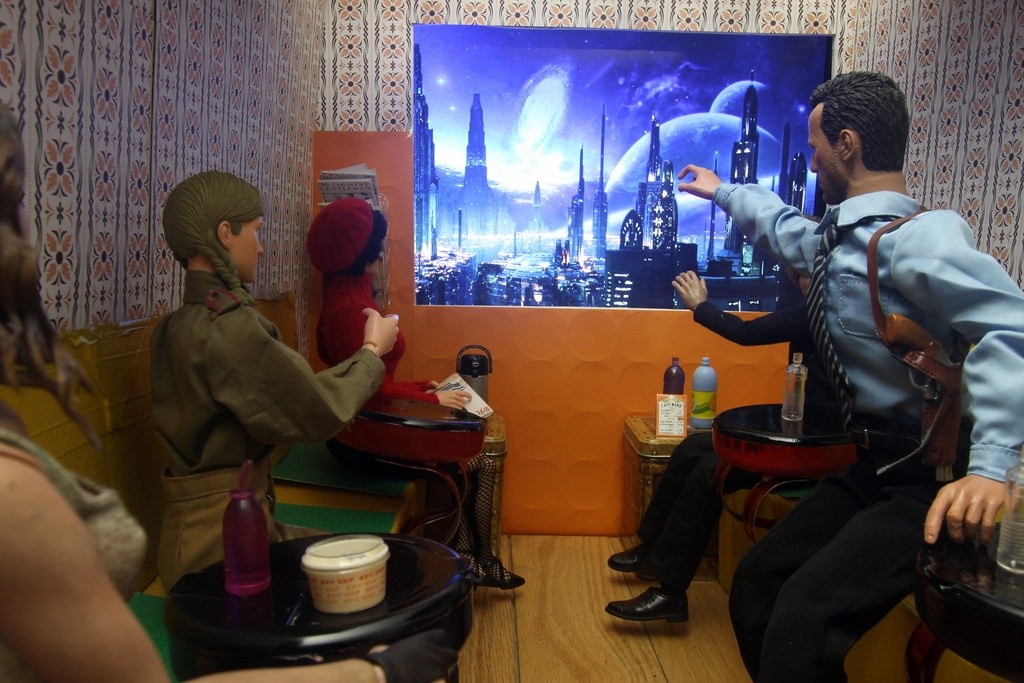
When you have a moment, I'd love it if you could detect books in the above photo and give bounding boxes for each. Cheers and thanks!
[316,162,387,309]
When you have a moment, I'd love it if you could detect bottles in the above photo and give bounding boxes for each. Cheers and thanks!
[997,445,1024,574]
[663,357,684,395]
[781,353,808,420]
[692,357,717,427]
[222,488,270,596]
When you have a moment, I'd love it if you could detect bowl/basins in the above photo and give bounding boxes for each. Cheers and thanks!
[300,532,390,614]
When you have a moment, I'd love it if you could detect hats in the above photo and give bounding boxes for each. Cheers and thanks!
[307,198,373,274]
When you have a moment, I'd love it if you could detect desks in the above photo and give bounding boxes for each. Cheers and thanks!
[333,399,486,545]
[626,411,719,580]
[902,534,1024,683]
[715,398,860,543]
[481,412,508,555]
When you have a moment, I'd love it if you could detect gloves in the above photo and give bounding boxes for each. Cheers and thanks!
[367,630,458,683]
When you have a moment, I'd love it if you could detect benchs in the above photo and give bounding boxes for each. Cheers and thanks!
[717,481,1014,683]
[121,439,427,683]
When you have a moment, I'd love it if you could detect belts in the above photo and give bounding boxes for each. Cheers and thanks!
[847,420,921,456]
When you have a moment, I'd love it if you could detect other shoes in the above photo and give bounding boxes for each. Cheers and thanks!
[472,562,525,590]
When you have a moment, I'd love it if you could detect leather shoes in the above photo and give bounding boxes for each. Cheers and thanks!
[605,586,688,623]
[608,544,661,572]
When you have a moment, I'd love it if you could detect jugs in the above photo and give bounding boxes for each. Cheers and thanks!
[456,344,492,420]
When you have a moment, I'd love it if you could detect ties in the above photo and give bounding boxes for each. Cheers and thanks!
[807,215,899,404]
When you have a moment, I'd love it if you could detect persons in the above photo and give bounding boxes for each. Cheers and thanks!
[0,103,409,683]
[151,170,399,591]
[308,199,527,591]
[606,72,1024,683]
